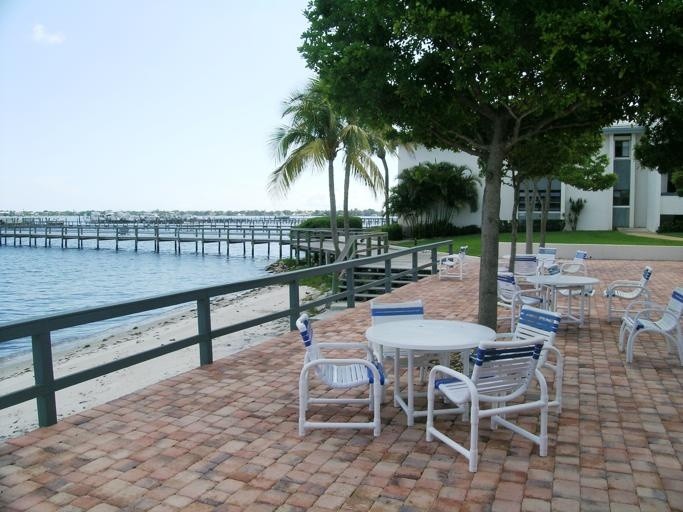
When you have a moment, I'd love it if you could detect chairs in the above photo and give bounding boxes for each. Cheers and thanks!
[500,246,591,291]
[487,304,569,423]
[602,264,654,326]
[435,246,474,281]
[494,272,545,331]
[617,286,681,368]
[424,336,553,473]
[364,298,452,406]
[555,263,596,316]
[294,312,385,440]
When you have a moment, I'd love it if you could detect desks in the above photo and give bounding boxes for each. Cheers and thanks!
[527,274,599,330]
[361,318,498,431]
